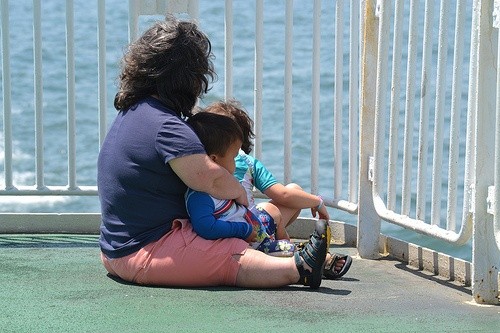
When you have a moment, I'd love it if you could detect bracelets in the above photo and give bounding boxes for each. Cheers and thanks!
[313,195,325,211]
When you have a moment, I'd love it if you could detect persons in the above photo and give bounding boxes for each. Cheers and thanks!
[204,97,329,229]
[184,108,352,280]
[96,18,331,289]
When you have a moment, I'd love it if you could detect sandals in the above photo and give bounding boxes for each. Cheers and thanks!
[323,250,353,279]
[293,218,331,290]
